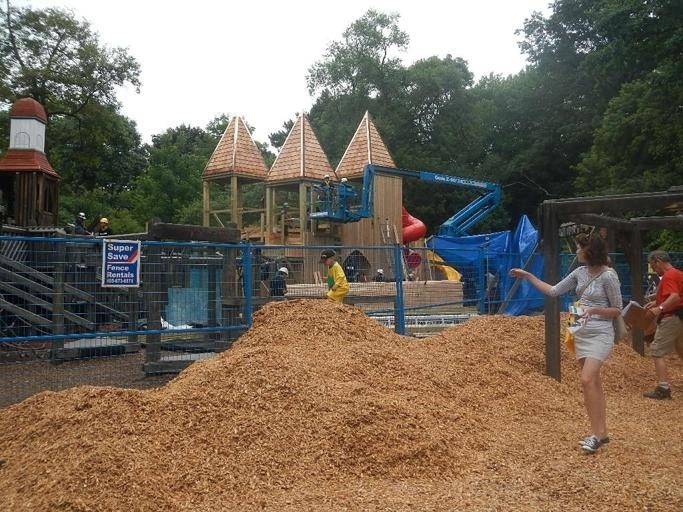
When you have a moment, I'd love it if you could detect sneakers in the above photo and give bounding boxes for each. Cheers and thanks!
[580,435,602,453]
[643,385,672,401]
[577,434,611,446]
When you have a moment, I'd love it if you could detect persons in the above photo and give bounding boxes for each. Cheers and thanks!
[339,177,350,210]
[269,267,288,296]
[372,268,389,282]
[281,202,293,245]
[72,212,88,265]
[509,233,623,452]
[319,249,349,303]
[643,250,683,400]
[314,175,335,211]
[94,218,114,265]
[256,256,288,288]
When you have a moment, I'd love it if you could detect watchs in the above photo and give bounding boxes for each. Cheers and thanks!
[658,304,663,312]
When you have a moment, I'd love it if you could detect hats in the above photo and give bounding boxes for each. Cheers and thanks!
[320,249,336,263]
[278,267,289,275]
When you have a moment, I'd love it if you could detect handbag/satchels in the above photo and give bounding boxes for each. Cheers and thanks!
[563,301,580,354]
[612,315,629,346]
